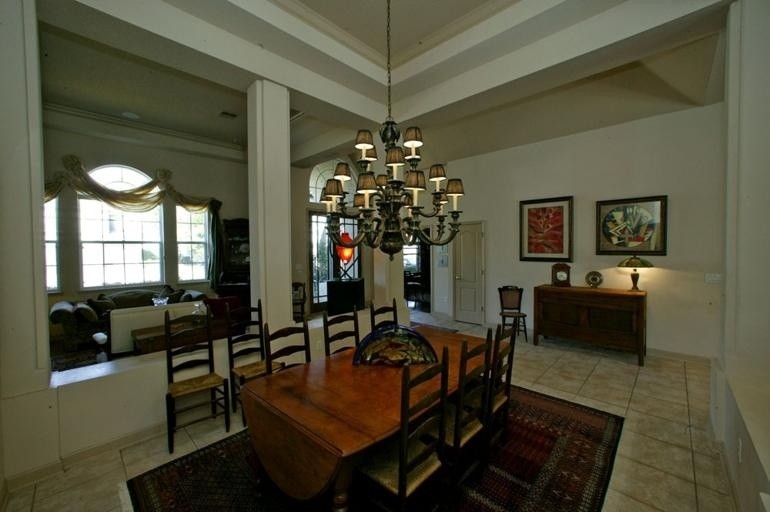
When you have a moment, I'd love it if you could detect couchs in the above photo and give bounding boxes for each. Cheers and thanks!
[50,284,209,355]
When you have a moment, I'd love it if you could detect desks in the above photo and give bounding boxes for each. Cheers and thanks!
[240,323,510,512]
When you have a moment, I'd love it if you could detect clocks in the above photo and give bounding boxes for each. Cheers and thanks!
[552,264,571,287]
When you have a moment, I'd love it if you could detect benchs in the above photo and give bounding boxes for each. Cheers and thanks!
[131,325,166,355]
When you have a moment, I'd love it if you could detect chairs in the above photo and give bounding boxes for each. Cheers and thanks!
[291,281,306,323]
[225,298,283,429]
[497,285,528,343]
[425,318,520,480]
[352,344,450,512]
[262,299,399,379]
[166,303,229,454]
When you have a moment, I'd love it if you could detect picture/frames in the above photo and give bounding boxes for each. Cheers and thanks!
[520,196,667,263]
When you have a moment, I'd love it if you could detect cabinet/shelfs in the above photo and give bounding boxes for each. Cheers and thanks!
[110,302,208,353]
[533,284,648,367]
[217,217,251,322]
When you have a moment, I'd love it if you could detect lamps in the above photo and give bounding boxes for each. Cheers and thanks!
[320,0,465,262]
[617,256,655,292]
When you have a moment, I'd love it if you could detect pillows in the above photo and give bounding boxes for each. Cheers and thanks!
[88,293,118,319]
[158,284,185,304]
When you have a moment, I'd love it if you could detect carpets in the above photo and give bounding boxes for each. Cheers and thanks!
[124,377,625,512]
